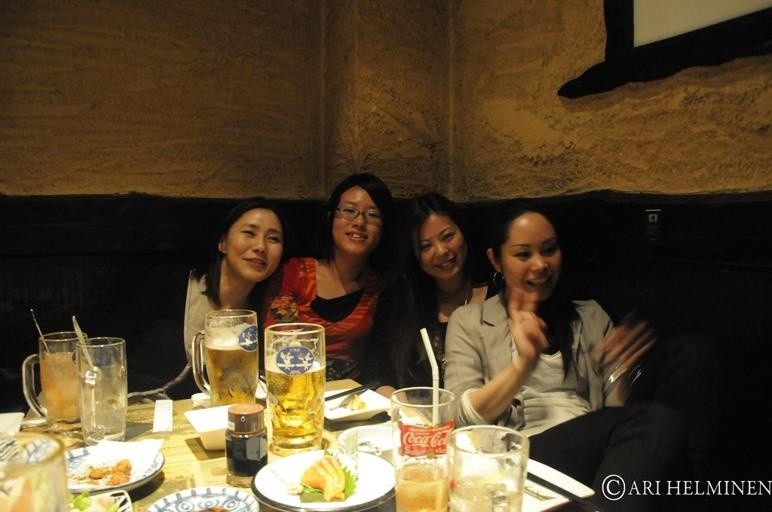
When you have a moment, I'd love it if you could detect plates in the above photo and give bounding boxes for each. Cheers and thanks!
[148,486,262,512]
[324,384,391,424]
[57,443,165,494]
[336,425,401,454]
[250,449,399,512]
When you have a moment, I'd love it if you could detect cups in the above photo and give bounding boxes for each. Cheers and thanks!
[389,386,456,512]
[20,330,89,427]
[444,424,530,512]
[77,336,129,447]
[0,429,71,512]
[263,322,325,456]
[189,308,260,414]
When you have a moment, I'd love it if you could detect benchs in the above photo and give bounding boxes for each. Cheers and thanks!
[0,186,772,512]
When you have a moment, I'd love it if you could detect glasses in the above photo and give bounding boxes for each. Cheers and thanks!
[333,206,388,227]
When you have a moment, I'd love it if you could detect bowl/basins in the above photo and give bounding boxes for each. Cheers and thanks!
[182,404,240,453]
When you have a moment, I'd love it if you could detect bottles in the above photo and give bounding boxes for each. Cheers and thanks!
[223,402,270,489]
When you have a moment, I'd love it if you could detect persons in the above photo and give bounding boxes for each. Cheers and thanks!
[88,198,288,404]
[363,193,499,402]
[443,206,677,512]
[262,171,396,386]
